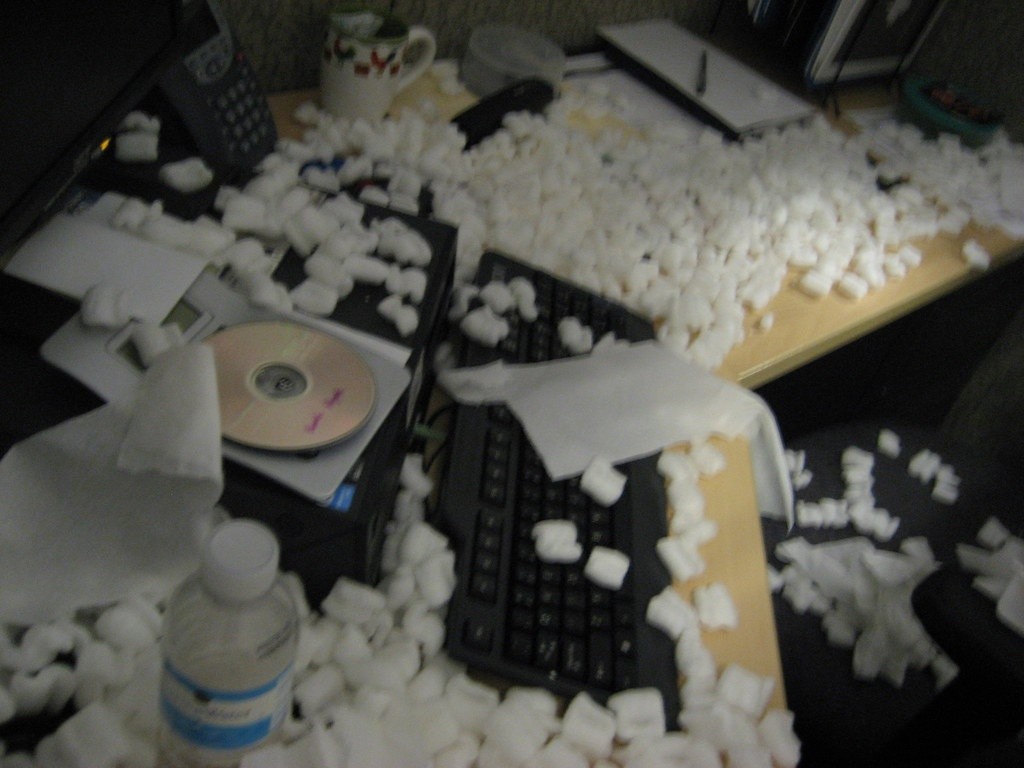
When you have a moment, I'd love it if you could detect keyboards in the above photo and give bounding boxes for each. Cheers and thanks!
[433,250,693,733]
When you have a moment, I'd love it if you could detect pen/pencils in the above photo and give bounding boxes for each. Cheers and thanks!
[696,49,707,93]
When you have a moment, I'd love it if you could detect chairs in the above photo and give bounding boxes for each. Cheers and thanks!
[760,307,1024,768]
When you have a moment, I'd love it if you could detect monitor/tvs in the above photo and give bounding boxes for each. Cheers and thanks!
[0,0,190,271]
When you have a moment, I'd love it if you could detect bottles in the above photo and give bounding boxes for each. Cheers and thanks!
[159,520,298,768]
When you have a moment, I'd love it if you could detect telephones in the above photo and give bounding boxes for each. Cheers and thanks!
[155,0,279,183]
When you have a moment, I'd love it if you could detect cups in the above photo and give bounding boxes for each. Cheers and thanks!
[318,17,435,127]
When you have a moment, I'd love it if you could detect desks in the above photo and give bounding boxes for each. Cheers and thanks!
[268,70,1024,767]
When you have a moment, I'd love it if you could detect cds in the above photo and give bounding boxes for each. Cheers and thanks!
[192,320,378,451]
